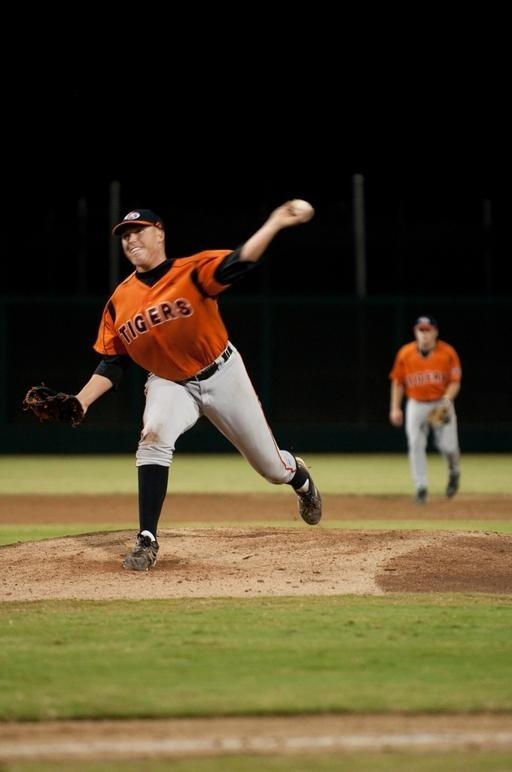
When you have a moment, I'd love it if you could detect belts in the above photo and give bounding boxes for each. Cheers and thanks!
[185,345,232,381]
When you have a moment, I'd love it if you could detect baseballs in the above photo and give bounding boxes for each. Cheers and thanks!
[291,200,311,214]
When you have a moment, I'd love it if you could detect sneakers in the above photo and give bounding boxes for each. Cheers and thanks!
[294,457,322,524]
[446,474,459,496]
[123,533,159,571]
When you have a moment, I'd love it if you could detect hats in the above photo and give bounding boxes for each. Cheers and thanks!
[112,209,162,235]
[415,316,437,327]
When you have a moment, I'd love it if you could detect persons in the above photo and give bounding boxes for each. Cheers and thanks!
[388,316,462,502]
[24,199,322,571]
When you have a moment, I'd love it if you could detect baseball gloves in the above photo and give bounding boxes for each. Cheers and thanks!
[25,387,83,426]
